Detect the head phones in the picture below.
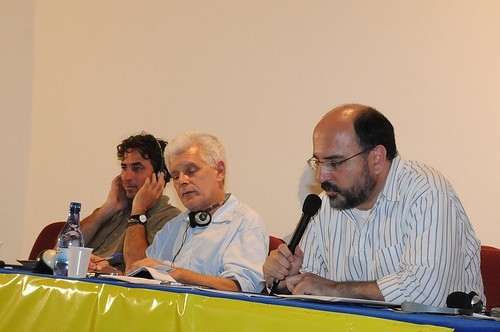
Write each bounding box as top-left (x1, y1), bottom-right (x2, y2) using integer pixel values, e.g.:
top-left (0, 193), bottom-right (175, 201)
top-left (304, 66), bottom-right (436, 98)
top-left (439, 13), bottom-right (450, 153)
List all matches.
top-left (186, 195), bottom-right (228, 228)
top-left (446, 291), bottom-right (484, 313)
top-left (156, 139), bottom-right (168, 180)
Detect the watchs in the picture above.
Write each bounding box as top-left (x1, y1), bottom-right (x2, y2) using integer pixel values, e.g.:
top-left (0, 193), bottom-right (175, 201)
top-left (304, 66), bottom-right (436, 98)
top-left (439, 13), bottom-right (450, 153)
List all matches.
top-left (129, 214), bottom-right (147, 223)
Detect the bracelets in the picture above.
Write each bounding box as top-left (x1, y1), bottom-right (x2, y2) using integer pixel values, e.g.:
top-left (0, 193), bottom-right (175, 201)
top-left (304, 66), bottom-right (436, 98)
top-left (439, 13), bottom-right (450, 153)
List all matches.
top-left (126, 222), bottom-right (146, 227)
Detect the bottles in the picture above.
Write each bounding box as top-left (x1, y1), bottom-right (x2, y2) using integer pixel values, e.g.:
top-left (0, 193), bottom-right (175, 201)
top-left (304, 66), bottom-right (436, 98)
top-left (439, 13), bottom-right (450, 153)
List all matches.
top-left (54, 202), bottom-right (86, 276)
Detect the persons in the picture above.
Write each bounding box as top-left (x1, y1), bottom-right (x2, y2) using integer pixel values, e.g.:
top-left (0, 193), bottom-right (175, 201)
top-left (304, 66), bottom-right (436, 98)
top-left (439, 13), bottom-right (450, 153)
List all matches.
top-left (53, 134), bottom-right (182, 272)
top-left (88, 131), bottom-right (270, 294)
top-left (262, 103), bottom-right (487, 310)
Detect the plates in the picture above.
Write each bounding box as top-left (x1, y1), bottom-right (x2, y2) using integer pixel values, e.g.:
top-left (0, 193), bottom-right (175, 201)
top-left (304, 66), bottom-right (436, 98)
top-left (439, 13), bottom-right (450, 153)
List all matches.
top-left (16, 260), bottom-right (36, 268)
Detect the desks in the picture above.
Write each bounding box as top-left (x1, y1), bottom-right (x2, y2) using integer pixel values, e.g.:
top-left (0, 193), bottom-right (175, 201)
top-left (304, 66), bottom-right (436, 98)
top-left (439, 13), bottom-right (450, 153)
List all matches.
top-left (0, 264), bottom-right (500, 332)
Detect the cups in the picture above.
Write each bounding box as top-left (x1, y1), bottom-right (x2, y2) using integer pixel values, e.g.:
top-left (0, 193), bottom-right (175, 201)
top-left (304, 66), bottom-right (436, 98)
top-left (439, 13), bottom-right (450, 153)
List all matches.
top-left (68, 246), bottom-right (94, 278)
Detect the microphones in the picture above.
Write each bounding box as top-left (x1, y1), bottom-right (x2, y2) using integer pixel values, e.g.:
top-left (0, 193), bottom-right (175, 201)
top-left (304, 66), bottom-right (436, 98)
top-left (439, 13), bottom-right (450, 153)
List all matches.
top-left (271, 194), bottom-right (322, 294)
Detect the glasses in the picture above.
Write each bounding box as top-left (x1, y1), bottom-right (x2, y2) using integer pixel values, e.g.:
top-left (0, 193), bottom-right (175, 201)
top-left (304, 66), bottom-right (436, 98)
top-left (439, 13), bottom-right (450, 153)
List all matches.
top-left (307, 146), bottom-right (376, 173)
